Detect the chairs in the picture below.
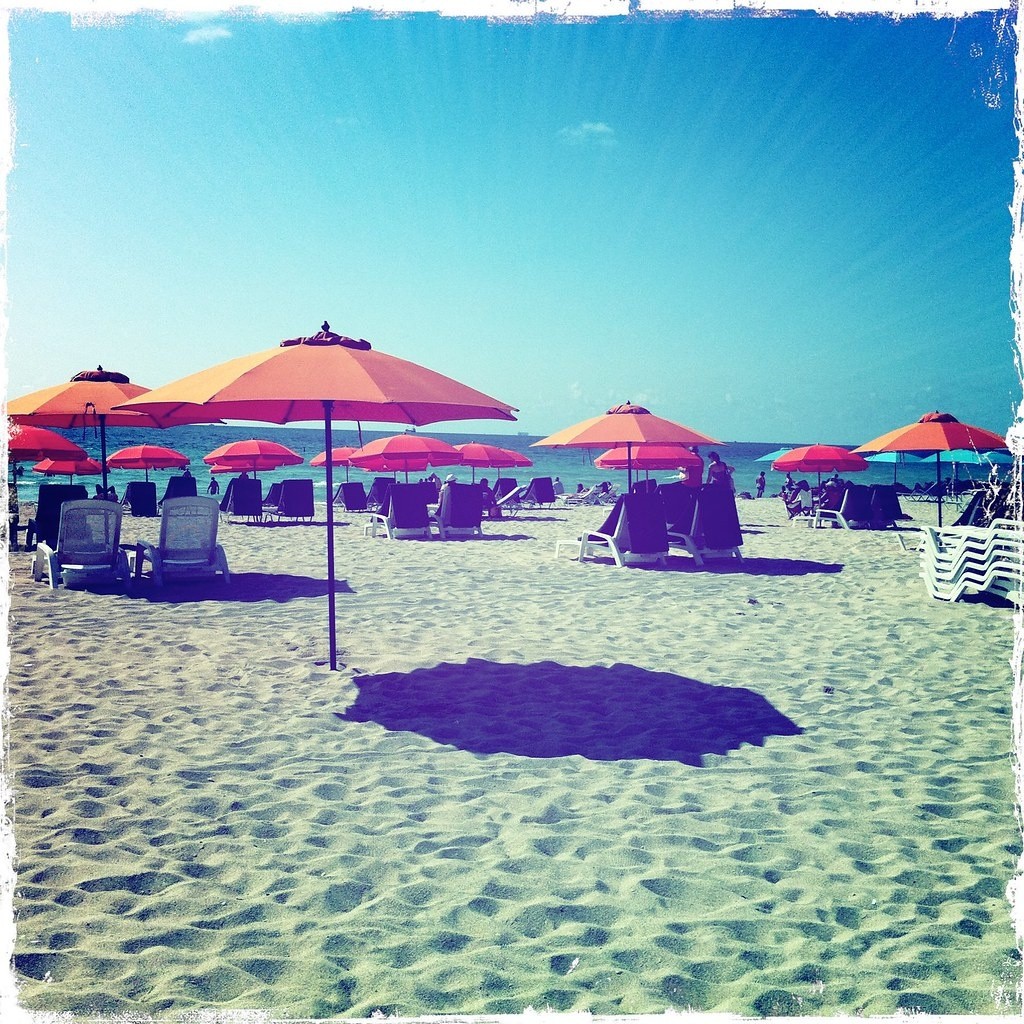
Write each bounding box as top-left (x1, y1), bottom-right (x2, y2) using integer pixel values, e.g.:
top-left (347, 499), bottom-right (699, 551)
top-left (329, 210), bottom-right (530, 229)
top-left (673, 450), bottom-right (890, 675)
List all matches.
top-left (261, 482), bottom-right (282, 507)
top-left (136, 496), bottom-right (231, 589)
top-left (792, 485), bottom-right (871, 528)
top-left (666, 483), bottom-right (745, 568)
top-left (364, 477), bottom-right (396, 512)
top-left (837, 485), bottom-right (897, 527)
top-left (427, 480), bottom-right (484, 540)
top-left (261, 478), bottom-right (315, 524)
top-left (895, 477), bottom-right (993, 512)
top-left (918, 518), bottom-right (1024, 607)
top-left (332, 482), bottom-right (369, 512)
top-left (482, 477), bottom-right (621, 519)
top-left (631, 479), bottom-right (657, 494)
top-left (34, 499), bottom-right (132, 592)
top-left (419, 482), bottom-right (438, 504)
top-left (119, 475), bottom-right (197, 517)
top-left (219, 478), bottom-right (262, 525)
top-left (25, 485), bottom-right (93, 552)
top-left (361, 482), bottom-right (439, 540)
top-left (555, 492), bottom-right (669, 569)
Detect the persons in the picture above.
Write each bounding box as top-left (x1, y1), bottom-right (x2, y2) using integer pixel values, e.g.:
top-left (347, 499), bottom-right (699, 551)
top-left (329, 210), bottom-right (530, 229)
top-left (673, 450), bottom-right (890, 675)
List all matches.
top-left (182, 467), bottom-right (191, 477)
top-left (756, 470), bottom-right (848, 528)
top-left (107, 486), bottom-right (118, 502)
top-left (682, 445), bottom-right (706, 488)
top-left (93, 484), bottom-right (105, 500)
top-left (239, 472), bottom-right (249, 479)
top-left (418, 472), bottom-right (612, 520)
top-left (705, 452), bottom-right (734, 484)
top-left (206, 477), bottom-right (220, 496)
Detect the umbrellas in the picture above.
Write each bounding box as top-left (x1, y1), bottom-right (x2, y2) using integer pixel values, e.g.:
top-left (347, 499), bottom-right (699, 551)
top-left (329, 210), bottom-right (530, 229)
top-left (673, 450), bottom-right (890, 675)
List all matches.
top-left (530, 402), bottom-right (729, 497)
top-left (772, 443), bottom-right (867, 510)
top-left (752, 446), bottom-right (798, 462)
top-left (8, 424), bottom-right (533, 496)
top-left (111, 319), bottom-right (519, 672)
top-left (594, 446), bottom-right (700, 495)
top-left (849, 411), bottom-right (1013, 527)
top-left (7, 363), bottom-right (226, 498)
